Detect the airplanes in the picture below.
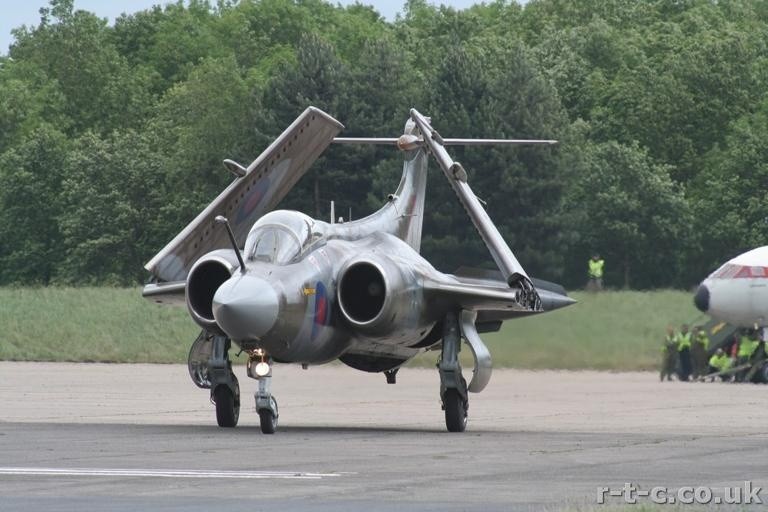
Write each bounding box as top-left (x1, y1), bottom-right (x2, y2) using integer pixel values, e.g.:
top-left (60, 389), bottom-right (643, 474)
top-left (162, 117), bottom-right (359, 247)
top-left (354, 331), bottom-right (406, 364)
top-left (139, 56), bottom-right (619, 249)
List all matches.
top-left (141, 105), bottom-right (580, 431)
top-left (690, 245), bottom-right (768, 327)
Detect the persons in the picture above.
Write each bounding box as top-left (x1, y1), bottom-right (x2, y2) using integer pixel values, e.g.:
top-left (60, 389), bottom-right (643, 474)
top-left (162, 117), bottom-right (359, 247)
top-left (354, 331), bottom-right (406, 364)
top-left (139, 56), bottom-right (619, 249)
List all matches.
top-left (587, 252), bottom-right (605, 290)
top-left (659, 323), bottom-right (767, 384)
